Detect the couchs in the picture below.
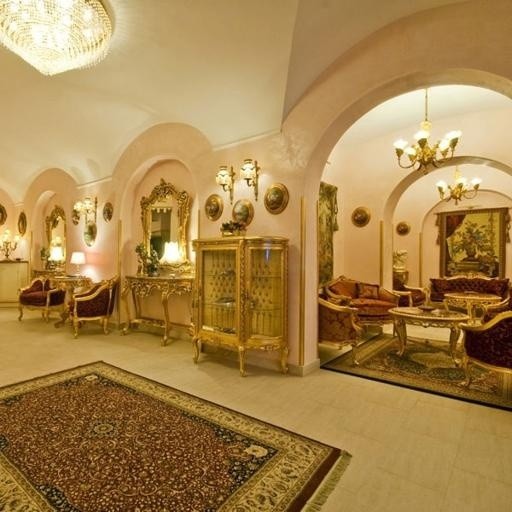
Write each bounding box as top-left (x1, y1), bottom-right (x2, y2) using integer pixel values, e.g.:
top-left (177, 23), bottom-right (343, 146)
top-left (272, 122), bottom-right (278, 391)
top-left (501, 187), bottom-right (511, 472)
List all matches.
top-left (17, 278), bottom-right (67, 324)
top-left (429, 275), bottom-right (510, 309)
top-left (318, 275), bottom-right (401, 367)
top-left (457, 311), bottom-right (512, 388)
top-left (393, 278), bottom-right (431, 307)
top-left (73, 274), bottom-right (119, 338)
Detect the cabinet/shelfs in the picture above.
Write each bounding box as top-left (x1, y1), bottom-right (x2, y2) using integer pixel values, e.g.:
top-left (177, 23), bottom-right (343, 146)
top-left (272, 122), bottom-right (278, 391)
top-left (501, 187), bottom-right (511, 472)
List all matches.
top-left (192, 236), bottom-right (289, 377)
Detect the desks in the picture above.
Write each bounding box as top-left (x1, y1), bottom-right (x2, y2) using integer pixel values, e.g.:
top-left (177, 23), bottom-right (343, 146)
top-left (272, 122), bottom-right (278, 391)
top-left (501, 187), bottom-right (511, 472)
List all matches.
top-left (121, 273), bottom-right (195, 347)
top-left (54, 276), bottom-right (92, 328)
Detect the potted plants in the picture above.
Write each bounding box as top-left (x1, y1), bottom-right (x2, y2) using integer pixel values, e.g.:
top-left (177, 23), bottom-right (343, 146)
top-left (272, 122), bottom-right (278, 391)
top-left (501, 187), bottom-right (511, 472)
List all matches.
top-left (135, 243), bottom-right (161, 276)
top-left (221, 219), bottom-right (247, 236)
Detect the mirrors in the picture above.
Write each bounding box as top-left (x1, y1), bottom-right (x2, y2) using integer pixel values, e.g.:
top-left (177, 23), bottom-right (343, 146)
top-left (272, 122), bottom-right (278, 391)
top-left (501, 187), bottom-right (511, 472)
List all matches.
top-left (140, 177), bottom-right (193, 274)
top-left (45, 204), bottom-right (67, 265)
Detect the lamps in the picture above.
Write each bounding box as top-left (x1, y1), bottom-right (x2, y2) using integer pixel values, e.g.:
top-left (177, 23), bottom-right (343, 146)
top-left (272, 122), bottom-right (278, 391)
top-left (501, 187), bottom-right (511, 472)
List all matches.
top-left (70, 251), bottom-right (86, 274)
top-left (392, 89), bottom-right (462, 175)
top-left (436, 165), bottom-right (482, 205)
top-left (74, 197), bottom-right (95, 224)
top-left (216, 165), bottom-right (236, 206)
top-left (0, 228), bottom-right (19, 260)
top-left (0, 0), bottom-right (113, 77)
top-left (240, 159), bottom-right (261, 202)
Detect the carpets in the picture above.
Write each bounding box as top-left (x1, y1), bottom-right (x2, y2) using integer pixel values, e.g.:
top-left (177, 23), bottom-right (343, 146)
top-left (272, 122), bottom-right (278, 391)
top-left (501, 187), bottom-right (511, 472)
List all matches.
top-left (320, 333), bottom-right (512, 412)
top-left (0, 360), bottom-right (353, 512)
